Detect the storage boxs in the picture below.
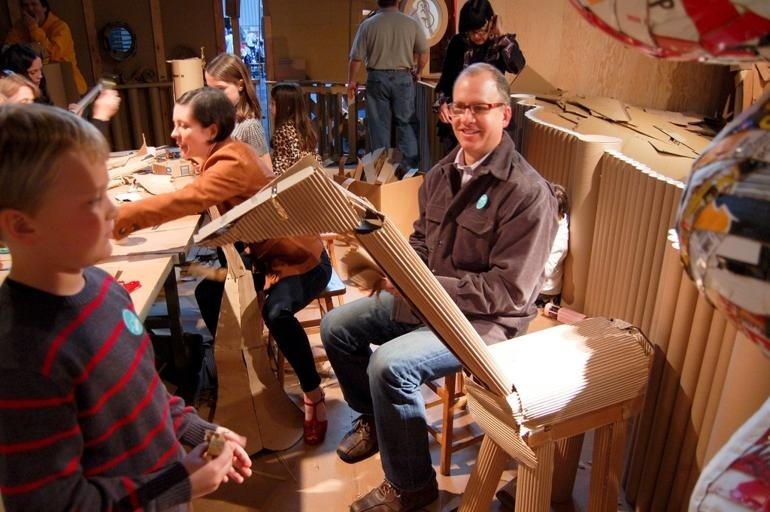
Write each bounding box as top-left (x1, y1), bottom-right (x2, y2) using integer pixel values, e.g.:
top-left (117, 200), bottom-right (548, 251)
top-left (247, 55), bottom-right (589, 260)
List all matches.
top-left (332, 165), bottom-right (423, 241)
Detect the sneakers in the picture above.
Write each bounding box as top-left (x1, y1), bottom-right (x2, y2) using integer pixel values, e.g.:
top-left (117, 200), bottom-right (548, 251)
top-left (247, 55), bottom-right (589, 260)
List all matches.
top-left (349, 475), bottom-right (439, 512)
top-left (337, 413), bottom-right (379, 464)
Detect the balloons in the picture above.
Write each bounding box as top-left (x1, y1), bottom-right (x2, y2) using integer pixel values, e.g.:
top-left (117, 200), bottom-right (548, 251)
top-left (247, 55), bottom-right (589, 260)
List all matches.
top-left (572, 1), bottom-right (770, 66)
top-left (687, 396), bottom-right (770, 512)
top-left (672, 83), bottom-right (770, 362)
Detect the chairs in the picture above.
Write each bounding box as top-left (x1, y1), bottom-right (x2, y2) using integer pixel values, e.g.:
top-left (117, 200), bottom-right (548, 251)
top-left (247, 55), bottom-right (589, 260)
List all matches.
top-left (209, 205), bottom-right (346, 392)
top-left (420, 181), bottom-right (569, 476)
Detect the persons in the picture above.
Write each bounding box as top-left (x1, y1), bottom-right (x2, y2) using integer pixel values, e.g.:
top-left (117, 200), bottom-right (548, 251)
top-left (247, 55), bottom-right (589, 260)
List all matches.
top-left (346, 0), bottom-right (428, 172)
top-left (0, 102), bottom-right (252, 510)
top-left (432, 0), bottom-right (525, 159)
top-left (319, 63), bottom-right (558, 512)
top-left (268, 83), bottom-right (318, 175)
top-left (205, 52), bottom-right (273, 174)
top-left (533, 183), bottom-right (569, 309)
top-left (0, 43), bottom-right (53, 105)
top-left (112, 86), bottom-right (331, 446)
top-left (0, 67), bottom-right (122, 146)
top-left (5, 0), bottom-right (88, 99)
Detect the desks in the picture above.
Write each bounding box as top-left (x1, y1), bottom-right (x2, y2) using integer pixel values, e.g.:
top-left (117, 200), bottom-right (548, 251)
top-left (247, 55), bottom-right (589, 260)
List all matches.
top-left (108, 150), bottom-right (206, 266)
top-left (0, 255), bottom-right (195, 406)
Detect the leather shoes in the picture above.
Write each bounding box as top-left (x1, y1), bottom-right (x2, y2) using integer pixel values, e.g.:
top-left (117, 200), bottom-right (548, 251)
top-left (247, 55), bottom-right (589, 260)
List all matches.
top-left (299, 386), bottom-right (328, 442)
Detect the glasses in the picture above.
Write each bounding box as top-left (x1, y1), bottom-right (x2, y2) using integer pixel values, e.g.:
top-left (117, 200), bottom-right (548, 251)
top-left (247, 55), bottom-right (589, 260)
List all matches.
top-left (447, 103), bottom-right (506, 120)
top-left (465, 21), bottom-right (490, 39)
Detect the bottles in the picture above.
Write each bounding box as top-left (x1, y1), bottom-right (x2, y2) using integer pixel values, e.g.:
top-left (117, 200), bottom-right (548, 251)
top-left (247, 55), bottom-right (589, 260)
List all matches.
top-left (543, 302), bottom-right (588, 324)
